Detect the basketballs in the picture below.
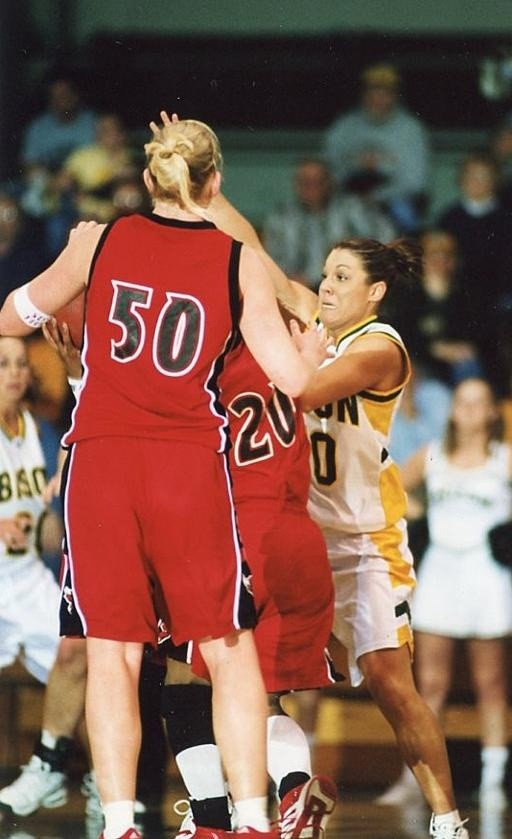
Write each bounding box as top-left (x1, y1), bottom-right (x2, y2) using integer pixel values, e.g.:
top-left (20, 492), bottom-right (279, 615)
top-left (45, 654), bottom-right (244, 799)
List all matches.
top-left (45, 287), bottom-right (87, 353)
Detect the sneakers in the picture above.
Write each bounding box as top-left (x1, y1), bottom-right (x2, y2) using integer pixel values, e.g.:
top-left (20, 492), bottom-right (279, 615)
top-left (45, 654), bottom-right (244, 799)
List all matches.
top-left (0, 755), bottom-right (70, 818)
top-left (429, 819), bottom-right (469, 839)
top-left (376, 784), bottom-right (423, 808)
top-left (173, 773), bottom-right (339, 839)
top-left (82, 771), bottom-right (148, 838)
top-left (479, 788), bottom-right (507, 812)
